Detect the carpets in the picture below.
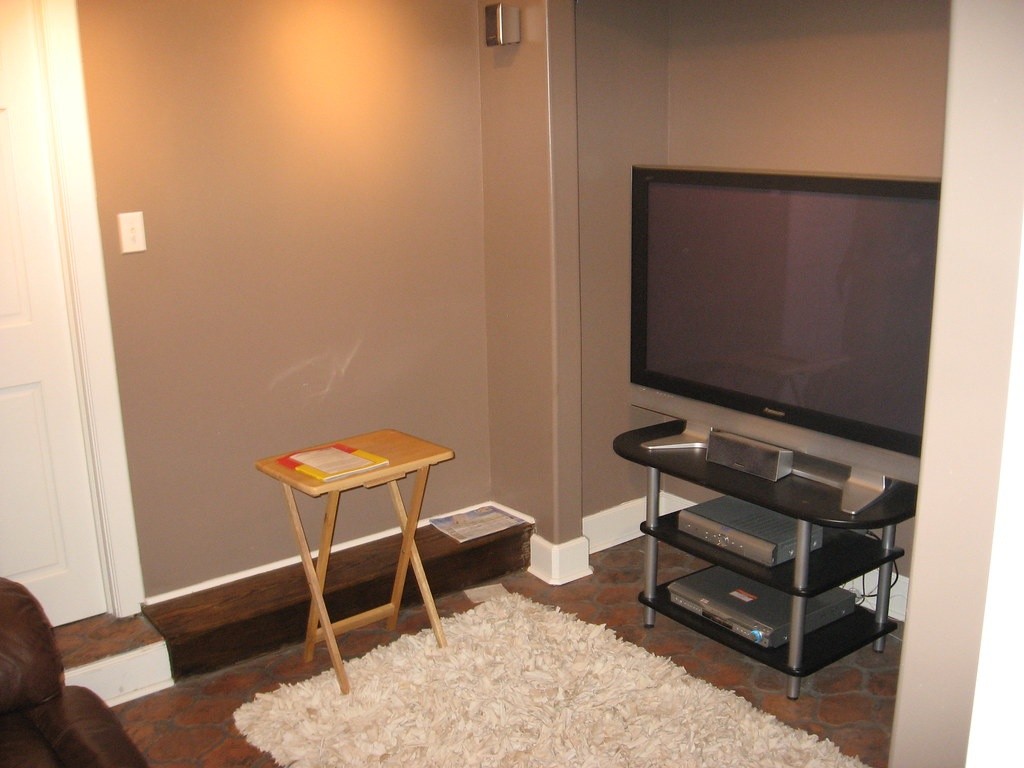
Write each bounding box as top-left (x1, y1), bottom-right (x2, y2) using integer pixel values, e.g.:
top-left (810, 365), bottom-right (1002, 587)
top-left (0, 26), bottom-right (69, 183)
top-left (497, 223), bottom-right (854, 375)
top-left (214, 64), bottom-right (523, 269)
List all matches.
top-left (232, 591), bottom-right (870, 768)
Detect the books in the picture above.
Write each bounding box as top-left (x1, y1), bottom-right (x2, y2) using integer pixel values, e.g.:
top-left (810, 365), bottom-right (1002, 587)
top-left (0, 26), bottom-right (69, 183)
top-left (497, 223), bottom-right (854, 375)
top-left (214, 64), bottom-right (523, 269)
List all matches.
top-left (277, 443), bottom-right (389, 484)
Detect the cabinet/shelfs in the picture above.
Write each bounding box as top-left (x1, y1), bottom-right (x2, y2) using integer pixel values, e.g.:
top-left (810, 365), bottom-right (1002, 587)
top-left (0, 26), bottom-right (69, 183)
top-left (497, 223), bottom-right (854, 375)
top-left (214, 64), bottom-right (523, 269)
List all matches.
top-left (613, 419), bottom-right (918, 702)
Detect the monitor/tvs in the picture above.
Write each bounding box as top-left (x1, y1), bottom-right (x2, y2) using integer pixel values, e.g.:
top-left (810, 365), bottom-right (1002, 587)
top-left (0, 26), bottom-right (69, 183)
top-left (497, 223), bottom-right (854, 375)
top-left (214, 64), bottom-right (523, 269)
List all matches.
top-left (629, 167), bottom-right (942, 515)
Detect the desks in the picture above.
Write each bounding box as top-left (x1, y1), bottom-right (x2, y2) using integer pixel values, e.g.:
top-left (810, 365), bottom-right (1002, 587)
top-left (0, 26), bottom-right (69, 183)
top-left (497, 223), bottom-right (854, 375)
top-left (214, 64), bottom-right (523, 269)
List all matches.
top-left (255, 428), bottom-right (455, 694)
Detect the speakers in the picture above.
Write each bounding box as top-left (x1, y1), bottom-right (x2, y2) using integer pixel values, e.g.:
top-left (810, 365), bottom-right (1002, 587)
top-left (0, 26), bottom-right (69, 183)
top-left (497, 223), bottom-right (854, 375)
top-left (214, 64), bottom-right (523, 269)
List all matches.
top-left (485, 3), bottom-right (520, 45)
top-left (705, 431), bottom-right (793, 482)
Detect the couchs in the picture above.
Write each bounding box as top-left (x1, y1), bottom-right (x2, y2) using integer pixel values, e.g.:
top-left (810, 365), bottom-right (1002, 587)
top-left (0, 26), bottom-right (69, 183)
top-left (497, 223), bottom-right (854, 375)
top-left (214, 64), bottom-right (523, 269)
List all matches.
top-left (0, 577), bottom-right (148, 768)
